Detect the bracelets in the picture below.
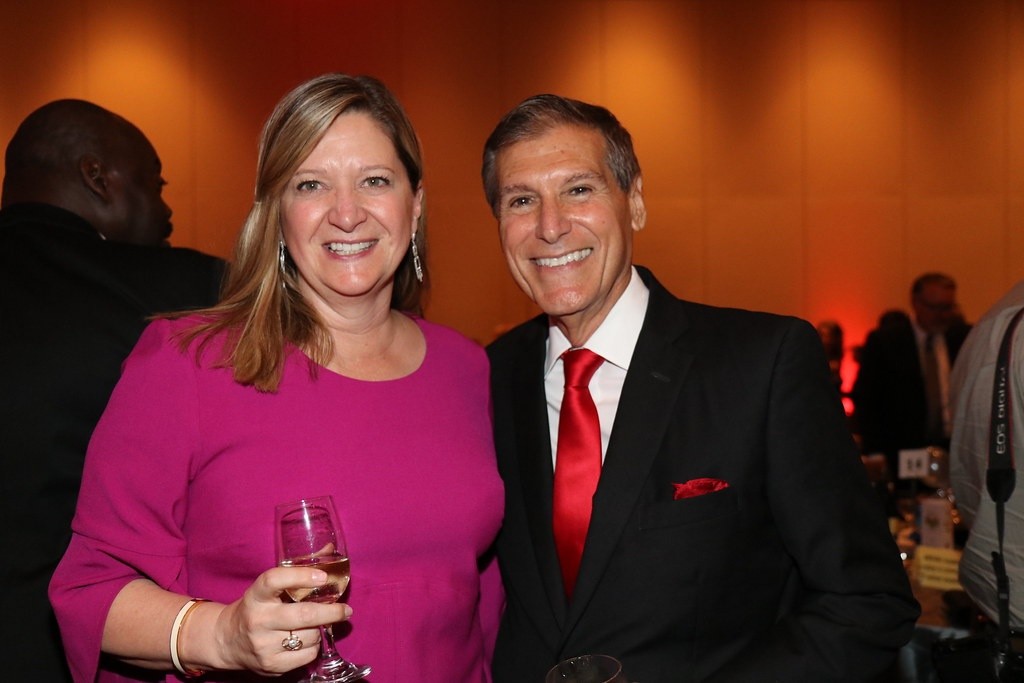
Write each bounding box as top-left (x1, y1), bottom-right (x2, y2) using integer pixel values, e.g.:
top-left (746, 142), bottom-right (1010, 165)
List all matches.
top-left (170, 598), bottom-right (213, 677)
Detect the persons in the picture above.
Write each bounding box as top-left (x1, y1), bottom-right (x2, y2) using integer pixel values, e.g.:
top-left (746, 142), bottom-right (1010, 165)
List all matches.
top-left (948, 281), bottom-right (1024, 683)
top-left (484, 93), bottom-right (921, 683)
top-left (0, 99), bottom-right (231, 683)
top-left (817, 271), bottom-right (973, 498)
top-left (48, 70), bottom-right (505, 683)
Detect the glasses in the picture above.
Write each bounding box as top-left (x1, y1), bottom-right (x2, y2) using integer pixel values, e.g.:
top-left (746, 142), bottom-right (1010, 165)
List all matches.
top-left (918, 298), bottom-right (957, 314)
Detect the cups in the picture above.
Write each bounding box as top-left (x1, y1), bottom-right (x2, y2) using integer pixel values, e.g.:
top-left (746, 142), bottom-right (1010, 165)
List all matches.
top-left (547, 654), bottom-right (622, 683)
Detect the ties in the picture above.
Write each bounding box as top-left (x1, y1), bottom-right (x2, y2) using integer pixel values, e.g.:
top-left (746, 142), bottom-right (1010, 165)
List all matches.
top-left (552, 348), bottom-right (606, 600)
top-left (925, 335), bottom-right (944, 424)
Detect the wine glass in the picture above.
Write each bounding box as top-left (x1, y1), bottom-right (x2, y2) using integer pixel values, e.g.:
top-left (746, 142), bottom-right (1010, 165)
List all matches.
top-left (275, 495), bottom-right (371, 682)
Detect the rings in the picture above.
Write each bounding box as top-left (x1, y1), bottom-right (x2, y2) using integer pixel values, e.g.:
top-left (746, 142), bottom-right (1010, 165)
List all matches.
top-left (282, 630), bottom-right (303, 651)
